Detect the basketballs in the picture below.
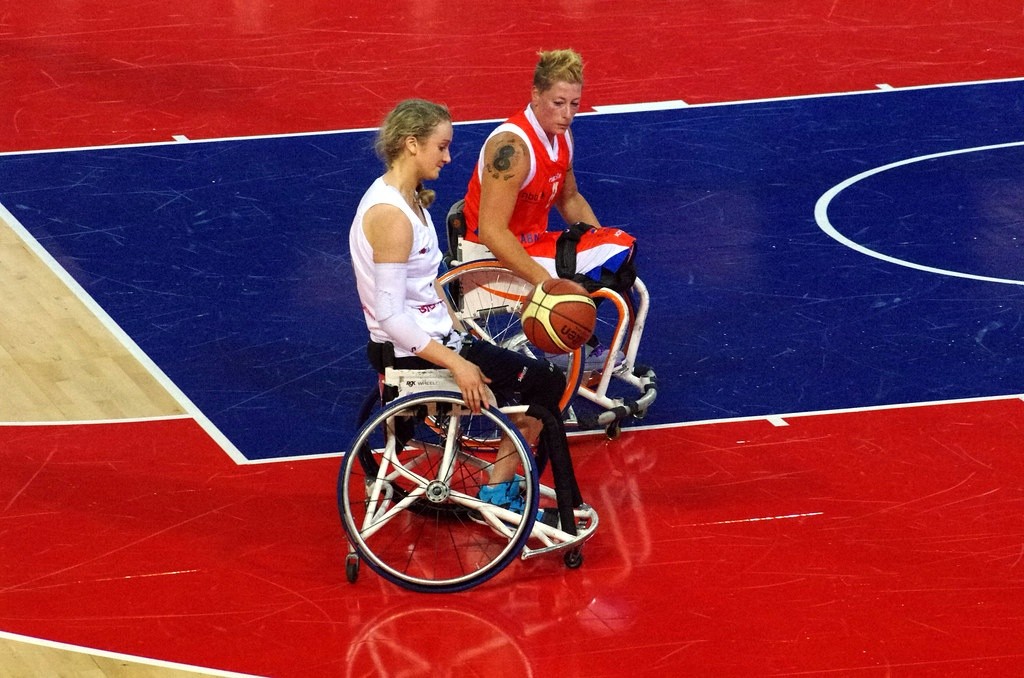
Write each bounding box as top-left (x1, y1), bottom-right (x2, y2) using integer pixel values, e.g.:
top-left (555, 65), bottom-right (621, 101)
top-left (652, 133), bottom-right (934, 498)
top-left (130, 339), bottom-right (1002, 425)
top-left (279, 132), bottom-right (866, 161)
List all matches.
top-left (520, 278), bottom-right (597, 354)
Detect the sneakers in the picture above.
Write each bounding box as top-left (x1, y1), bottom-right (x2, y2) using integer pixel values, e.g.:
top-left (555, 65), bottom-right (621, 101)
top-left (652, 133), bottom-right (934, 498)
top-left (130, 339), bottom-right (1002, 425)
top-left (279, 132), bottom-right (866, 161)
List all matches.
top-left (469, 475), bottom-right (549, 527)
top-left (544, 335), bottom-right (628, 372)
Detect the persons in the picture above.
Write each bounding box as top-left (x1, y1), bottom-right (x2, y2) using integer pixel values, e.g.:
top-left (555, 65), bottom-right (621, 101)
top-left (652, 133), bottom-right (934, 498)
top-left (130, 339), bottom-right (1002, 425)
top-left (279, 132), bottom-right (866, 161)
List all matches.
top-left (348, 100), bottom-right (566, 534)
top-left (461, 48), bottom-right (636, 371)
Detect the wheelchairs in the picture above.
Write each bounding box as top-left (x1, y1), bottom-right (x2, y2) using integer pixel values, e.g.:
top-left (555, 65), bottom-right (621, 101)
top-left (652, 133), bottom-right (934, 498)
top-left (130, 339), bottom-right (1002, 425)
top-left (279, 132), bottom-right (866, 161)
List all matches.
top-left (437, 200), bottom-right (660, 439)
top-left (336, 257), bottom-right (599, 594)
